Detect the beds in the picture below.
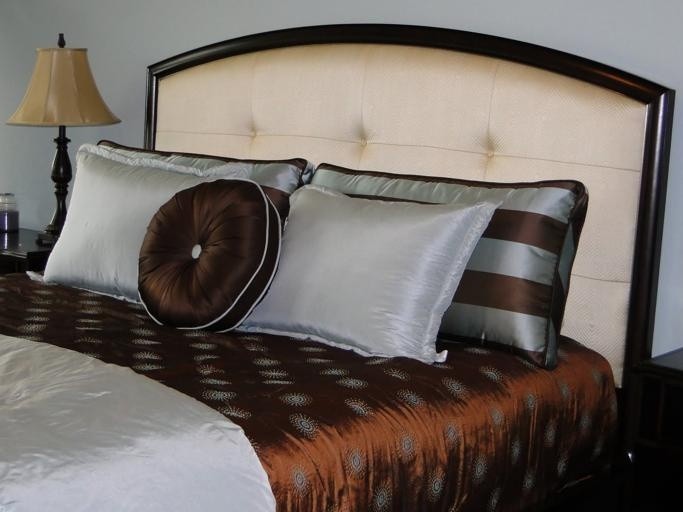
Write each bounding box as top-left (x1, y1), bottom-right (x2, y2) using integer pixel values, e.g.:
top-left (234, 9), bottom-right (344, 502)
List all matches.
top-left (0, 23), bottom-right (678, 511)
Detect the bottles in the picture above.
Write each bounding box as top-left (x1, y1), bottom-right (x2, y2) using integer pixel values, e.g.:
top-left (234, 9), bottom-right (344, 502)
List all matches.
top-left (0, 231), bottom-right (20, 253)
top-left (0, 192), bottom-right (19, 234)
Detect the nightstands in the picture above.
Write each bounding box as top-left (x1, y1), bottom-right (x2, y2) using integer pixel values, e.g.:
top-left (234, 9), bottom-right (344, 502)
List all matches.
top-left (0, 228), bottom-right (56, 273)
top-left (610, 348), bottom-right (682, 512)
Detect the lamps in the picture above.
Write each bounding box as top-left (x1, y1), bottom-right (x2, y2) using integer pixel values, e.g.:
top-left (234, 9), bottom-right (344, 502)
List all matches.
top-left (6, 30), bottom-right (120, 247)
